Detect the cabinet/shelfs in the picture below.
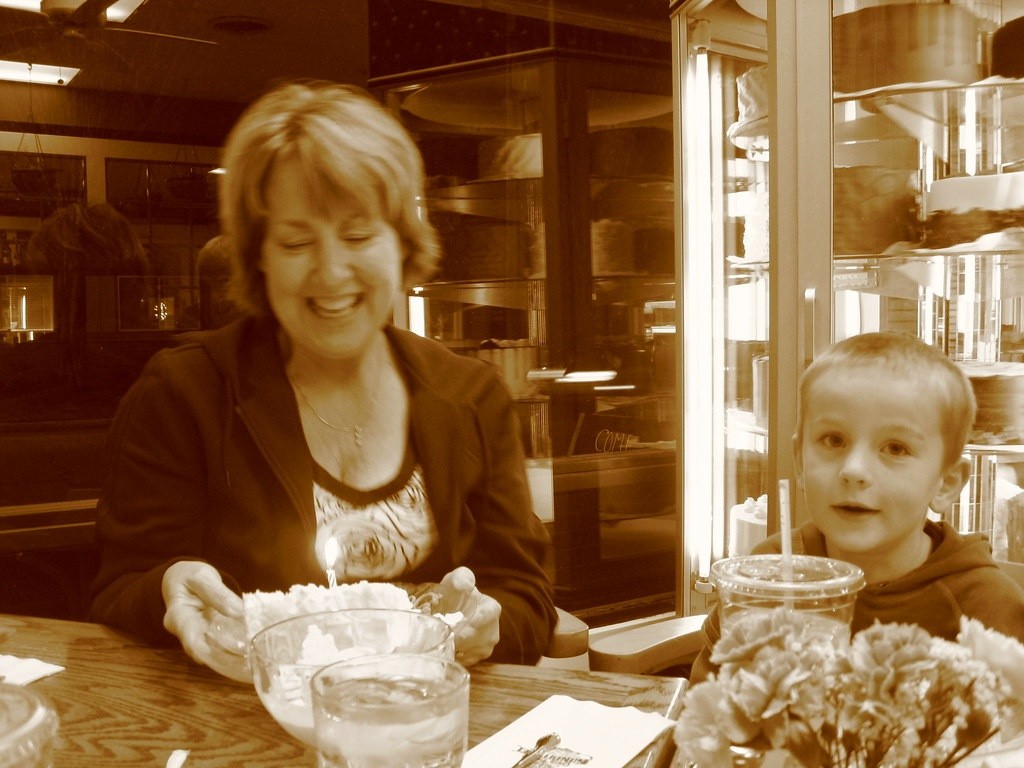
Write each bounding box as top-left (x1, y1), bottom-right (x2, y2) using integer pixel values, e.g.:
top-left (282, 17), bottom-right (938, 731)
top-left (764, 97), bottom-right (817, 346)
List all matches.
top-left (668, 2), bottom-right (1024, 608)
top-left (367, 45), bottom-right (670, 614)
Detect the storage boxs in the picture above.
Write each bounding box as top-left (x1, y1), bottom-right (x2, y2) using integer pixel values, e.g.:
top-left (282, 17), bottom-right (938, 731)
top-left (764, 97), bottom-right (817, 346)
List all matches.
top-left (835, 3), bottom-right (981, 93)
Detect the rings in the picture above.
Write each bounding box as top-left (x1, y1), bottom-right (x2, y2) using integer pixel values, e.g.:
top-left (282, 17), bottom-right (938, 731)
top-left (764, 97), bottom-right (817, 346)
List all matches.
top-left (456, 650), bottom-right (465, 658)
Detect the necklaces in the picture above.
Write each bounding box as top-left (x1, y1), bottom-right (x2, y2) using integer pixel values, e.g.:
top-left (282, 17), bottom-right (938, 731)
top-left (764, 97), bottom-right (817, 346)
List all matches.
top-left (289, 335), bottom-right (382, 447)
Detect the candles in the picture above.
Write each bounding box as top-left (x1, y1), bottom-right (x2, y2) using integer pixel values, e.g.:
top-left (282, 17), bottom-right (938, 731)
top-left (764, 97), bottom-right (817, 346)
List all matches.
top-left (325, 570), bottom-right (342, 610)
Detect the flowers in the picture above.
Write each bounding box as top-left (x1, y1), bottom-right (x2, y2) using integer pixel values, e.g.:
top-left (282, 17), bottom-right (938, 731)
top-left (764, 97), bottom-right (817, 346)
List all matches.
top-left (670, 609), bottom-right (1024, 768)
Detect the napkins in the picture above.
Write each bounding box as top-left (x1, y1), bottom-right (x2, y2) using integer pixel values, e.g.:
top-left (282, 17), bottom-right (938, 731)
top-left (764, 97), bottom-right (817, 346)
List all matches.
top-left (461, 695), bottom-right (677, 768)
top-left (0, 654), bottom-right (66, 686)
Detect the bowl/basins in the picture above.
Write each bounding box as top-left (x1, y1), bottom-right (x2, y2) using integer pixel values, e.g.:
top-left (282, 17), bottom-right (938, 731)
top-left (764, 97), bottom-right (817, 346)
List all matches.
top-left (247, 607), bottom-right (457, 748)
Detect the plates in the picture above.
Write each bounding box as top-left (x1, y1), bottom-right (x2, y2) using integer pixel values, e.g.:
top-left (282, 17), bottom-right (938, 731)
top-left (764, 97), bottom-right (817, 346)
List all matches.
top-left (201, 580), bottom-right (483, 686)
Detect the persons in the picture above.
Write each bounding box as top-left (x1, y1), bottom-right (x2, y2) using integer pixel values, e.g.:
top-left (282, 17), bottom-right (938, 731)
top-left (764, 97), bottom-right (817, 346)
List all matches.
top-left (689, 331), bottom-right (1024, 693)
top-left (171, 236), bottom-right (249, 345)
top-left (85, 85), bottom-right (556, 683)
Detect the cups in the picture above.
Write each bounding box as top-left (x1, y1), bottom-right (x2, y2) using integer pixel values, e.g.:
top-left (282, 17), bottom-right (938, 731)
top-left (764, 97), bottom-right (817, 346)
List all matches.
top-left (0, 683), bottom-right (64, 768)
top-left (309, 655), bottom-right (471, 768)
top-left (708, 555), bottom-right (867, 649)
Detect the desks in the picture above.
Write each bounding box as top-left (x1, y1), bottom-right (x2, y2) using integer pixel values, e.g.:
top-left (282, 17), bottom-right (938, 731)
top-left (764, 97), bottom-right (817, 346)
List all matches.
top-left (0, 615), bottom-right (693, 768)
